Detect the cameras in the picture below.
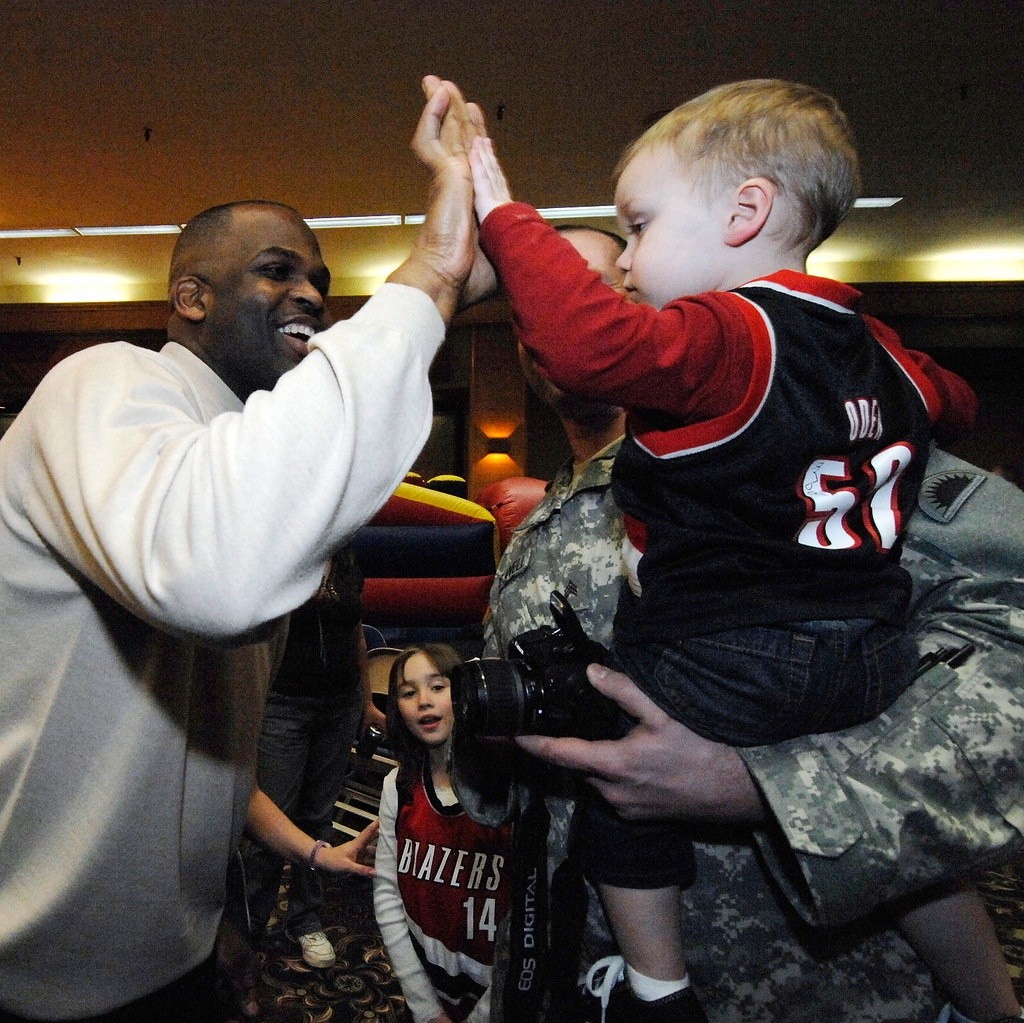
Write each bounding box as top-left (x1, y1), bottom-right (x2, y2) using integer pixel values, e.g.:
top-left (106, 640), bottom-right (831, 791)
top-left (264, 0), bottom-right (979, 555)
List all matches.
top-left (451, 590), bottom-right (629, 744)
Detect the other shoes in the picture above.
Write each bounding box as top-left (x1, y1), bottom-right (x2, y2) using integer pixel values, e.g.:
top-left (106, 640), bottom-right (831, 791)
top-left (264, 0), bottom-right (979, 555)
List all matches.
top-left (547, 952), bottom-right (709, 1023)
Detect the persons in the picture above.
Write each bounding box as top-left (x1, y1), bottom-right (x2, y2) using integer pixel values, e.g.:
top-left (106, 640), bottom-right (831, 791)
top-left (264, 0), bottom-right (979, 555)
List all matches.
top-left (468, 78), bottom-right (1024, 1023)
top-left (372, 642), bottom-right (515, 1023)
top-left (1, 75), bottom-right (498, 1023)
top-left (485, 223), bottom-right (1023, 1023)
top-left (244, 787), bottom-right (379, 879)
top-left (244, 548), bottom-right (385, 968)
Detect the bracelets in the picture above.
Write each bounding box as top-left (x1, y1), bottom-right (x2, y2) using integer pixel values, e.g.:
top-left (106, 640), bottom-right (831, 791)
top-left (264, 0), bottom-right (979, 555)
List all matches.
top-left (310, 840), bottom-right (331, 870)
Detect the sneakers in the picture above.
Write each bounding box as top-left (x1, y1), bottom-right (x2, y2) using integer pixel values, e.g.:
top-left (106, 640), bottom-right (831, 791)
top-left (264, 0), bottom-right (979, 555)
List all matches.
top-left (286, 924), bottom-right (336, 968)
